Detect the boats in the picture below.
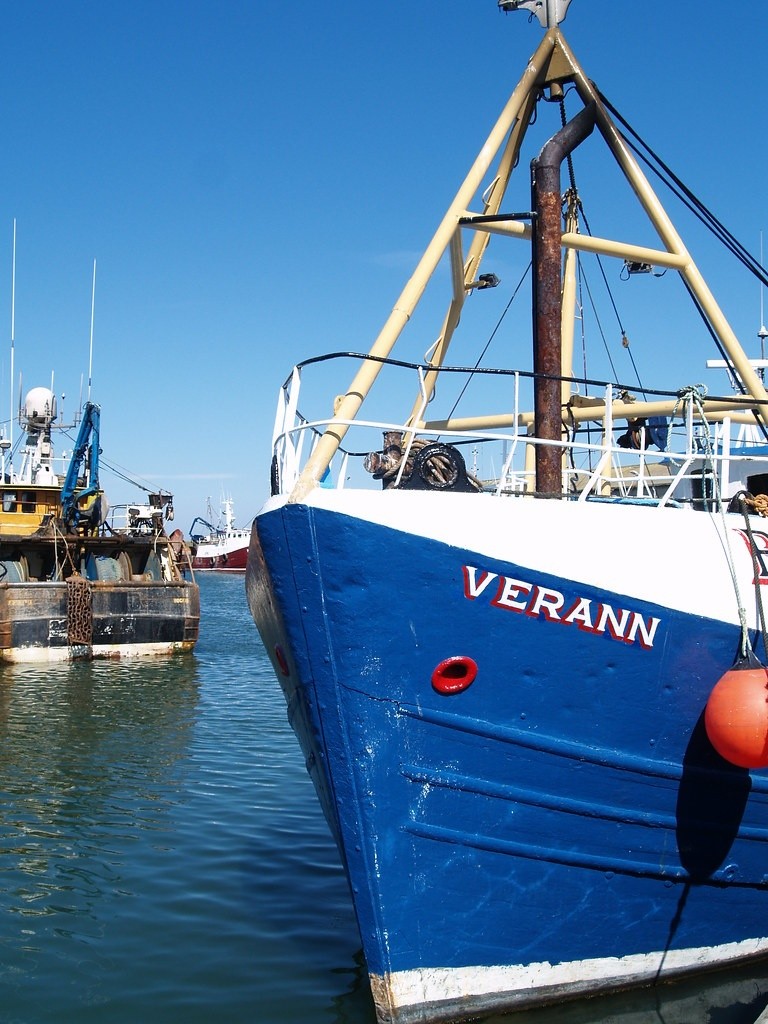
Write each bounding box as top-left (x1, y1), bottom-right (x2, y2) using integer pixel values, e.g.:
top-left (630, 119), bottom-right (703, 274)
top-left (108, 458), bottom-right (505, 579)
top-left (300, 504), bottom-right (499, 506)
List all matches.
top-left (245, 0), bottom-right (768, 1024)
top-left (0, 219), bottom-right (201, 663)
top-left (182, 494), bottom-right (251, 570)
